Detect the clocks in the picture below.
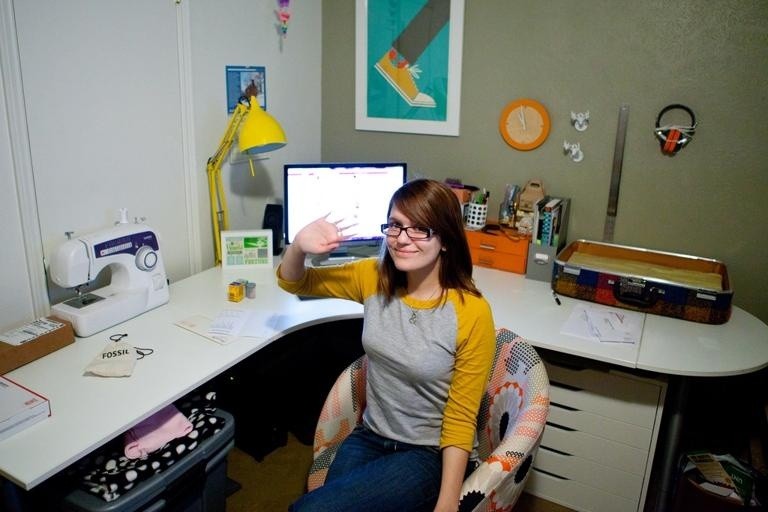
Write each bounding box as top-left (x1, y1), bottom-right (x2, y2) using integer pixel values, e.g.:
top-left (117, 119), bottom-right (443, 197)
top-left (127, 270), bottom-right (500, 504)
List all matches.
top-left (500, 99), bottom-right (550, 150)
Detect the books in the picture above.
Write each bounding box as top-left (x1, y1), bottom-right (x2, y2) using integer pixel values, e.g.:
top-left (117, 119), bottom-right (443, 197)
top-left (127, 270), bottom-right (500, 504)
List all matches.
top-left (533, 195), bottom-right (566, 246)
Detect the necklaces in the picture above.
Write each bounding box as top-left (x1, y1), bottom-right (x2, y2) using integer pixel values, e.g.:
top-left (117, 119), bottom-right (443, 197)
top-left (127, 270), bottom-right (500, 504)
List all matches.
top-left (397, 282), bottom-right (440, 323)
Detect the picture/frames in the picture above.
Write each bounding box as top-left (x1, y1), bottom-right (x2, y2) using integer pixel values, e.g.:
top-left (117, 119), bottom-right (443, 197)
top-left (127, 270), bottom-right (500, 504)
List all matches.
top-left (355, 0), bottom-right (464, 136)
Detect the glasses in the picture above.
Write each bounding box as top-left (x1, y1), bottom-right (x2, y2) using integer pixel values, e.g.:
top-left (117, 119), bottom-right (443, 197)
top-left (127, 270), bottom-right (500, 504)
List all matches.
top-left (378, 221), bottom-right (440, 241)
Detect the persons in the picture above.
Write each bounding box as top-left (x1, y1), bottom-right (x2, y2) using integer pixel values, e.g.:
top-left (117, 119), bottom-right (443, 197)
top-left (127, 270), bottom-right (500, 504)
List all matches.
top-left (274, 178), bottom-right (497, 512)
top-left (374, 0), bottom-right (450, 109)
top-left (244, 77), bottom-right (259, 98)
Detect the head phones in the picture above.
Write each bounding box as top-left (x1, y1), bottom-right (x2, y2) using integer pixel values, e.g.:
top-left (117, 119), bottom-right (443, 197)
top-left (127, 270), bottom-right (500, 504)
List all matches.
top-left (656, 104), bottom-right (696, 156)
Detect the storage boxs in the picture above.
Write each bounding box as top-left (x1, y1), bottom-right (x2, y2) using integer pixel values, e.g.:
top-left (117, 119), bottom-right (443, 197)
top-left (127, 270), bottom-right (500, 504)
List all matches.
top-left (50, 393), bottom-right (235, 512)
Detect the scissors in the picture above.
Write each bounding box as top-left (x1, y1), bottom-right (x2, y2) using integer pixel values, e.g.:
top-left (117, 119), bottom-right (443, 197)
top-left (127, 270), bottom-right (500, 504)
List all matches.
top-left (498, 222), bottom-right (527, 242)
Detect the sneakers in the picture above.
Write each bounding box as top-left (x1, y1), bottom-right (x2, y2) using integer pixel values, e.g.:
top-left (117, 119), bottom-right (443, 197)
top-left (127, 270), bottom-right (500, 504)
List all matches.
top-left (371, 43), bottom-right (441, 112)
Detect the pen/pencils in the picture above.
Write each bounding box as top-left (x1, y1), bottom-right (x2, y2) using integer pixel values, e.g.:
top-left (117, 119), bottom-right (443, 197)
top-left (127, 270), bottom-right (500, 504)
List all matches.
top-left (451, 185), bottom-right (480, 190)
top-left (552, 290), bottom-right (560, 305)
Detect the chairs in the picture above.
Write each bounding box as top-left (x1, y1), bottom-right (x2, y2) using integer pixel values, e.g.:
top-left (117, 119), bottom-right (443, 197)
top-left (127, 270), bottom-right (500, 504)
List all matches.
top-left (307, 328), bottom-right (550, 512)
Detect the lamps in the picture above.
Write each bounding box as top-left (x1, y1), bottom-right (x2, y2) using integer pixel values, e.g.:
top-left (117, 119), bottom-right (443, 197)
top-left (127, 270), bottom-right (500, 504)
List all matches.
top-left (206, 80), bottom-right (287, 268)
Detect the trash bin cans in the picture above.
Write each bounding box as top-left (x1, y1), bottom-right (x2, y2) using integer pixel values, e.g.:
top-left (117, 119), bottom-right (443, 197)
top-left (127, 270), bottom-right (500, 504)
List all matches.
top-left (668, 450), bottom-right (768, 511)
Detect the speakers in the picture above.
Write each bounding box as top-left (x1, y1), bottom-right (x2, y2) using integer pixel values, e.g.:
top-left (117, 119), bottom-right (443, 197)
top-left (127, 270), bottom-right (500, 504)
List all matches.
top-left (262, 204), bottom-right (283, 255)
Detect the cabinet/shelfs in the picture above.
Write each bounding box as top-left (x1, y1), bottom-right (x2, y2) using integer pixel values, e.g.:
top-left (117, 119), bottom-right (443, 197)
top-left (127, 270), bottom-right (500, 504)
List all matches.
top-left (522, 358), bottom-right (667, 511)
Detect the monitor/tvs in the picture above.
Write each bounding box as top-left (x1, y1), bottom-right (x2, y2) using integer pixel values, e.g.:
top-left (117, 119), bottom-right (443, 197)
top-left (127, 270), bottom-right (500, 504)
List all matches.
top-left (284, 162), bottom-right (407, 266)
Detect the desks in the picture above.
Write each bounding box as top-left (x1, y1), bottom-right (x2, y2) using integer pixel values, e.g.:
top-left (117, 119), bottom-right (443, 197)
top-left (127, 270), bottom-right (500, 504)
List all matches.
top-left (0, 258), bottom-right (767, 492)
